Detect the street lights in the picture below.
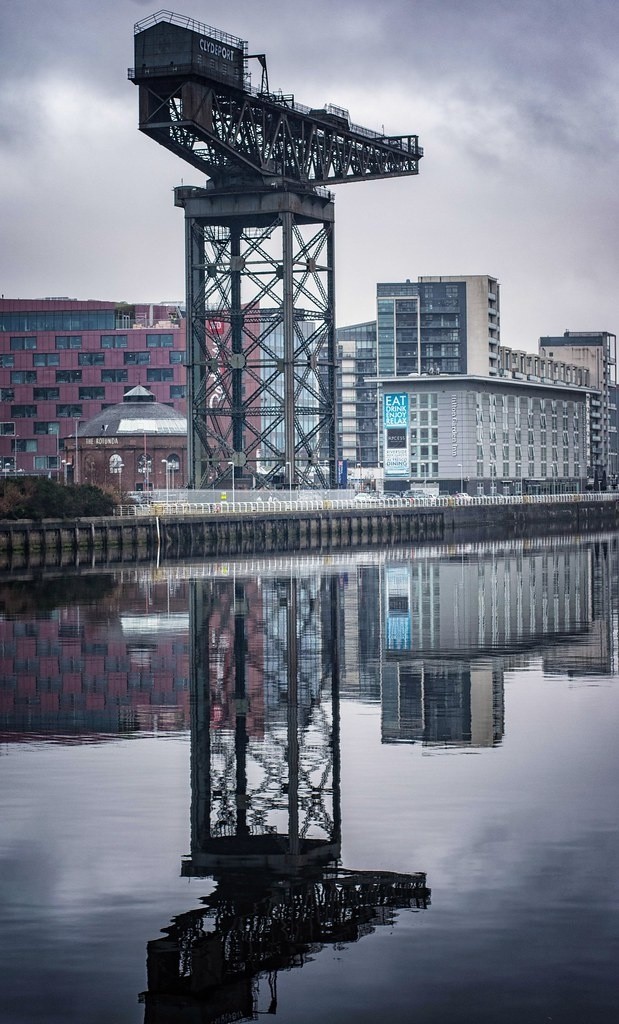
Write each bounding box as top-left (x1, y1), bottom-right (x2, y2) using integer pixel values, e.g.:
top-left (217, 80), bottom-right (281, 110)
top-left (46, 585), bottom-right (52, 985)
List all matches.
top-left (488, 464), bottom-right (494, 496)
top-left (61, 459), bottom-right (72, 487)
top-left (420, 462), bottom-right (428, 498)
top-left (517, 464), bottom-right (523, 496)
top-left (161, 458), bottom-right (170, 504)
top-left (118, 463), bottom-right (124, 491)
top-left (457, 464), bottom-right (464, 492)
top-left (286, 461), bottom-right (291, 501)
top-left (4, 463), bottom-right (10, 479)
top-left (578, 465), bottom-right (582, 494)
top-left (550, 465), bottom-right (554, 494)
top-left (357, 463), bottom-right (363, 493)
top-left (228, 461), bottom-right (235, 502)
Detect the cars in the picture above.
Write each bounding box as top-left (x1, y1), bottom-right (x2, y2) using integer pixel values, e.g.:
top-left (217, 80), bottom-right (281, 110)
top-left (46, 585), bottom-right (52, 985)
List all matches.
top-left (350, 487), bottom-right (505, 502)
